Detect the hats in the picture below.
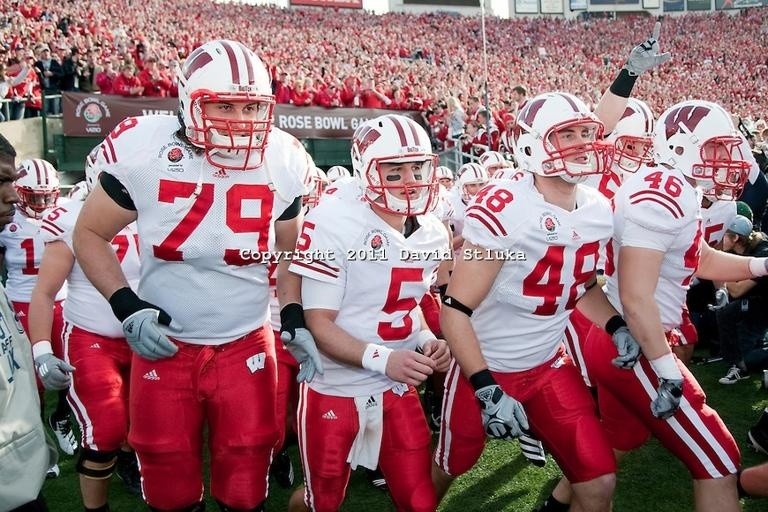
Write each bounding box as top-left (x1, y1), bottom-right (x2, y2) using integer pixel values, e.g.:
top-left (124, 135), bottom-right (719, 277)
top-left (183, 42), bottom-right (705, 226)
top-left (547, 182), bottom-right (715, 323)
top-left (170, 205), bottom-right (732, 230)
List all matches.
top-left (727, 214), bottom-right (753, 236)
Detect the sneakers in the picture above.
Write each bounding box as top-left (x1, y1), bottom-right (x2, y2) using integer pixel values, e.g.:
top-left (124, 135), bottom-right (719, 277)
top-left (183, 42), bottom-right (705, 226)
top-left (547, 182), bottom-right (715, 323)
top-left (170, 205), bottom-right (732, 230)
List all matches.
top-left (46, 464), bottom-right (59, 479)
top-left (275, 449), bottom-right (294, 488)
top-left (719, 364), bottom-right (752, 383)
top-left (50, 409), bottom-right (78, 456)
top-left (520, 429), bottom-right (548, 467)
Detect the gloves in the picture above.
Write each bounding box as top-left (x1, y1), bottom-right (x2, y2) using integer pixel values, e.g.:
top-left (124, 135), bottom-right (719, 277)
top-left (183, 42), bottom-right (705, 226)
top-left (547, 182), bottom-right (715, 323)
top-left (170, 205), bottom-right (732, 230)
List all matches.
top-left (278, 302), bottom-right (325, 383)
top-left (707, 287), bottom-right (729, 310)
top-left (606, 313), bottom-right (642, 371)
top-left (608, 21), bottom-right (670, 99)
top-left (651, 354), bottom-right (685, 419)
top-left (108, 287), bottom-right (183, 362)
top-left (751, 256), bottom-right (768, 276)
top-left (469, 368), bottom-right (530, 439)
top-left (31, 340), bottom-right (77, 395)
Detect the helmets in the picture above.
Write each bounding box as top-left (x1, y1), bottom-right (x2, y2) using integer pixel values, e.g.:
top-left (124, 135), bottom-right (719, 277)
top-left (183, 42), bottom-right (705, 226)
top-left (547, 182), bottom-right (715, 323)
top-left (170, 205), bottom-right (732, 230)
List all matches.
top-left (179, 40), bottom-right (273, 159)
top-left (649, 100), bottom-right (735, 182)
top-left (67, 182), bottom-right (87, 200)
top-left (351, 114), bottom-right (433, 215)
top-left (512, 92), bottom-right (602, 183)
top-left (456, 163), bottom-right (488, 185)
top-left (13, 159), bottom-right (60, 220)
top-left (434, 167), bottom-right (449, 180)
top-left (436, 166), bottom-right (453, 181)
top-left (608, 98), bottom-right (654, 168)
top-left (86, 141), bottom-right (106, 192)
top-left (328, 167), bottom-right (350, 183)
top-left (726, 131), bottom-right (760, 185)
top-left (478, 152), bottom-right (509, 168)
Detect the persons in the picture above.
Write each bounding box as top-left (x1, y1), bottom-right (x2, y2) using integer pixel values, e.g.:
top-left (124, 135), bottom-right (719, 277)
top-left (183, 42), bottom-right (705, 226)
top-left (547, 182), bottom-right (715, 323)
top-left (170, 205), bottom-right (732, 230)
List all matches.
top-left (0, 0), bottom-right (768, 147)
top-left (536, 99), bottom-right (768, 512)
top-left (268, 151), bottom-right (321, 490)
top-left (672, 128), bottom-right (760, 365)
top-left (432, 92), bottom-right (617, 512)
top-left (72, 39), bottom-right (323, 511)
top-left (28, 141), bottom-right (139, 511)
top-left (429, 182), bottom-right (453, 299)
top-left (1, 159), bottom-right (80, 457)
top-left (479, 150), bottom-right (506, 179)
top-left (435, 166), bottom-right (454, 189)
top-left (0, 132), bottom-right (58, 511)
top-left (593, 22), bottom-right (671, 210)
top-left (715, 214), bottom-right (768, 384)
top-left (448, 163), bottom-right (487, 277)
top-left (289, 115), bottom-right (452, 512)
